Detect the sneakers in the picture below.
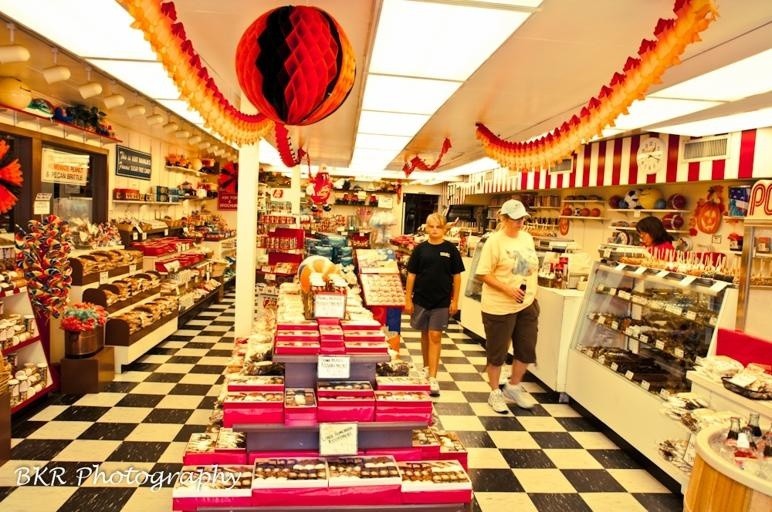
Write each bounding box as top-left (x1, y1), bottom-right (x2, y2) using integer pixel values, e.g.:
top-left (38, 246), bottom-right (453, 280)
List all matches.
top-left (488, 389), bottom-right (509, 414)
top-left (503, 380), bottom-right (534, 409)
top-left (421, 366), bottom-right (439, 394)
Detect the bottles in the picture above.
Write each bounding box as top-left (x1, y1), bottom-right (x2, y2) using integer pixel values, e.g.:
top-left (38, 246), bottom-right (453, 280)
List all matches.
top-left (727, 412), bottom-right (772, 469)
top-left (516, 278), bottom-right (526, 304)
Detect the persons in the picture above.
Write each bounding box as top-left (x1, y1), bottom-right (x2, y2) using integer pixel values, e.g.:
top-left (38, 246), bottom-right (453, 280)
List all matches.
top-left (404, 213), bottom-right (466, 392)
top-left (635, 216), bottom-right (678, 263)
top-left (474, 199), bottom-right (541, 414)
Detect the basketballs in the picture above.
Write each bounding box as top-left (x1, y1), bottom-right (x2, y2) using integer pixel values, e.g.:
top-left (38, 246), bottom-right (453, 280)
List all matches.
top-left (298, 255), bottom-right (339, 294)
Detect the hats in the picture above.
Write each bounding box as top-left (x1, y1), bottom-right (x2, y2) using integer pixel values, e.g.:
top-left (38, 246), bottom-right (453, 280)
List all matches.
top-left (499, 200), bottom-right (531, 220)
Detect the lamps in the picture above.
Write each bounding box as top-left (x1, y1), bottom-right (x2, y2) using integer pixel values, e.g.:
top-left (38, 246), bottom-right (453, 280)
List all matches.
top-left (348, 0), bottom-right (549, 172)
top-left (0, 0), bottom-right (239, 163)
